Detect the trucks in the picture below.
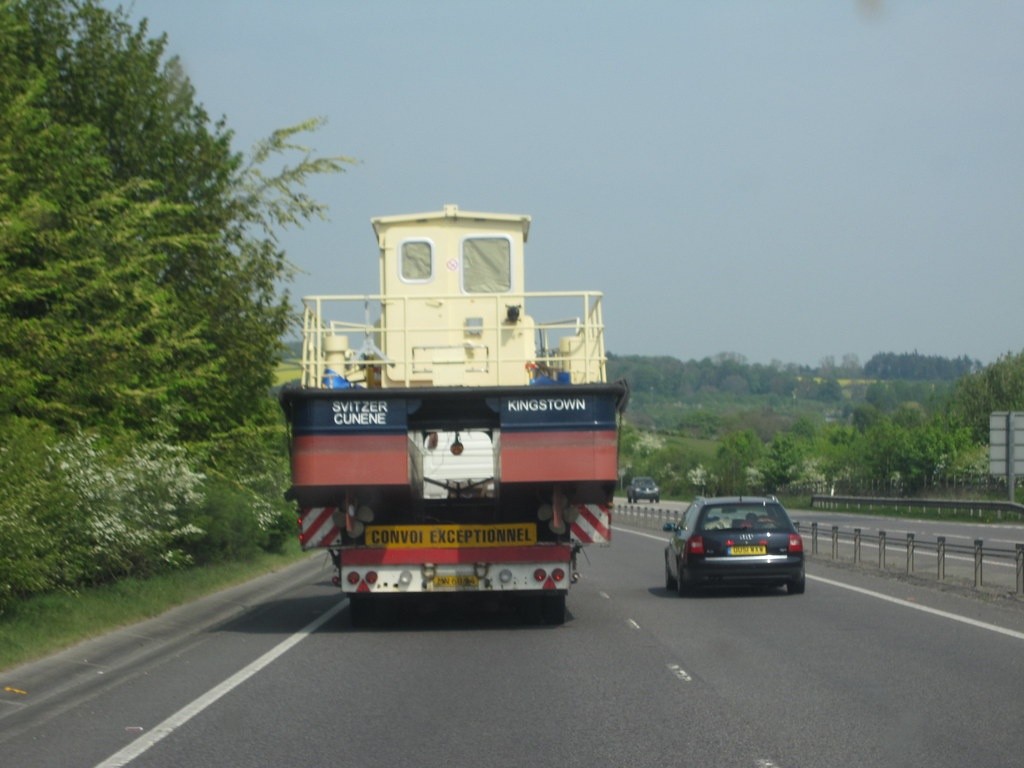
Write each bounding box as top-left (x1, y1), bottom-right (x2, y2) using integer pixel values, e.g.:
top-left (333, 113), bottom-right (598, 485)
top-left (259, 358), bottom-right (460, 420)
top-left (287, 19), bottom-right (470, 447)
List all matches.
top-left (280, 204), bottom-right (630, 624)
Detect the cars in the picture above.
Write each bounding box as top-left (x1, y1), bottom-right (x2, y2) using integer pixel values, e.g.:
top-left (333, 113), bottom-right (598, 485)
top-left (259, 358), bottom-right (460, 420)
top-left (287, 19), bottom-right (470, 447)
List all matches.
top-left (628, 476), bottom-right (661, 504)
top-left (661, 495), bottom-right (806, 596)
